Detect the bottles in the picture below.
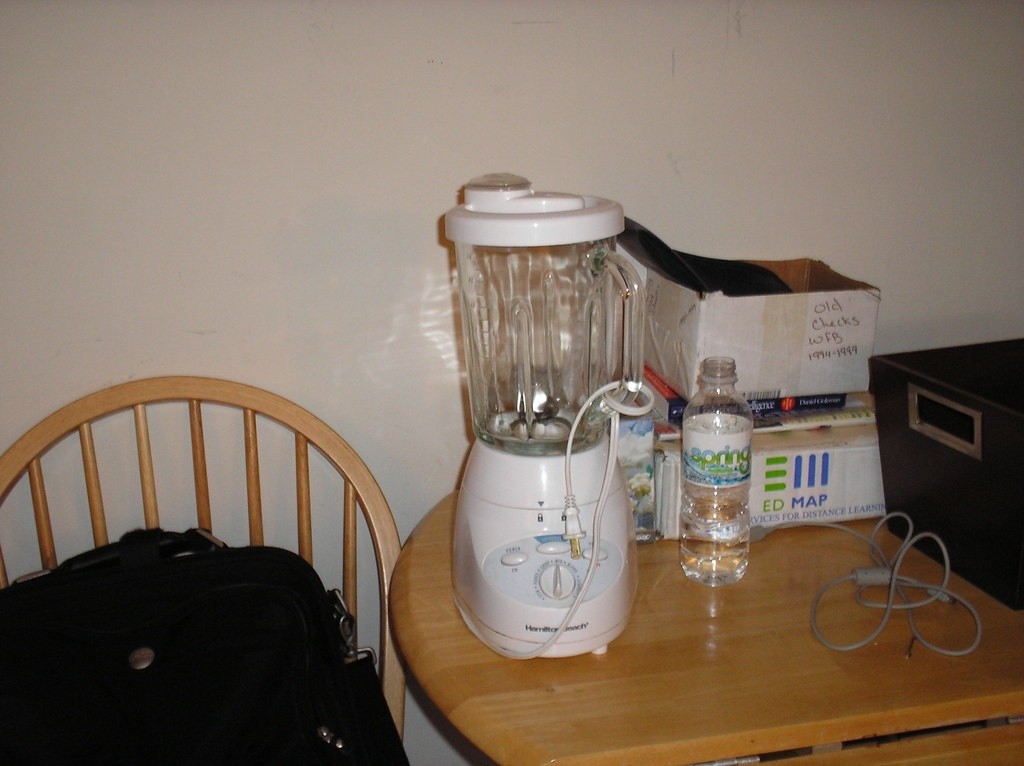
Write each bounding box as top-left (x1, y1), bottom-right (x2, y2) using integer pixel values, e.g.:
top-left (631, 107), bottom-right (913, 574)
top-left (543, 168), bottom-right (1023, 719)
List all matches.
top-left (681, 356), bottom-right (751, 588)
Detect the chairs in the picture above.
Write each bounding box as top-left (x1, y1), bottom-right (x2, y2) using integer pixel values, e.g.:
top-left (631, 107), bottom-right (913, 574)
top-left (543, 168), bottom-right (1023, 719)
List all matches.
top-left (0, 373), bottom-right (405, 750)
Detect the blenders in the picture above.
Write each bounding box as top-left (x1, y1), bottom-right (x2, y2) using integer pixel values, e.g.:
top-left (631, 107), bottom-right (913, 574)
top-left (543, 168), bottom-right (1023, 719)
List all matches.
top-left (444, 173), bottom-right (654, 659)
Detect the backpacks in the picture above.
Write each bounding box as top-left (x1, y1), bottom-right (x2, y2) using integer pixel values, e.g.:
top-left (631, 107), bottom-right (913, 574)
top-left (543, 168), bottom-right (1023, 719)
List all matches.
top-left (0, 528), bottom-right (410, 766)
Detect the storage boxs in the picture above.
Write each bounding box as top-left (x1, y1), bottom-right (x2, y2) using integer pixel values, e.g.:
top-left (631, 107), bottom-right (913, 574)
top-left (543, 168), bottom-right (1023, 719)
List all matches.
top-left (652, 424), bottom-right (887, 537)
top-left (634, 255), bottom-right (880, 402)
top-left (870, 337), bottom-right (1024, 613)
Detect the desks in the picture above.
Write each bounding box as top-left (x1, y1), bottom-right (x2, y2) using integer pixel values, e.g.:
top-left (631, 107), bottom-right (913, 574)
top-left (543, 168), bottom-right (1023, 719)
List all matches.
top-left (386, 483), bottom-right (1023, 765)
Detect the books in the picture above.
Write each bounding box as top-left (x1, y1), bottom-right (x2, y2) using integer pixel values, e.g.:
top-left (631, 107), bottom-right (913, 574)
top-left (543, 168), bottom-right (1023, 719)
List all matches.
top-left (643, 364), bottom-right (877, 441)
